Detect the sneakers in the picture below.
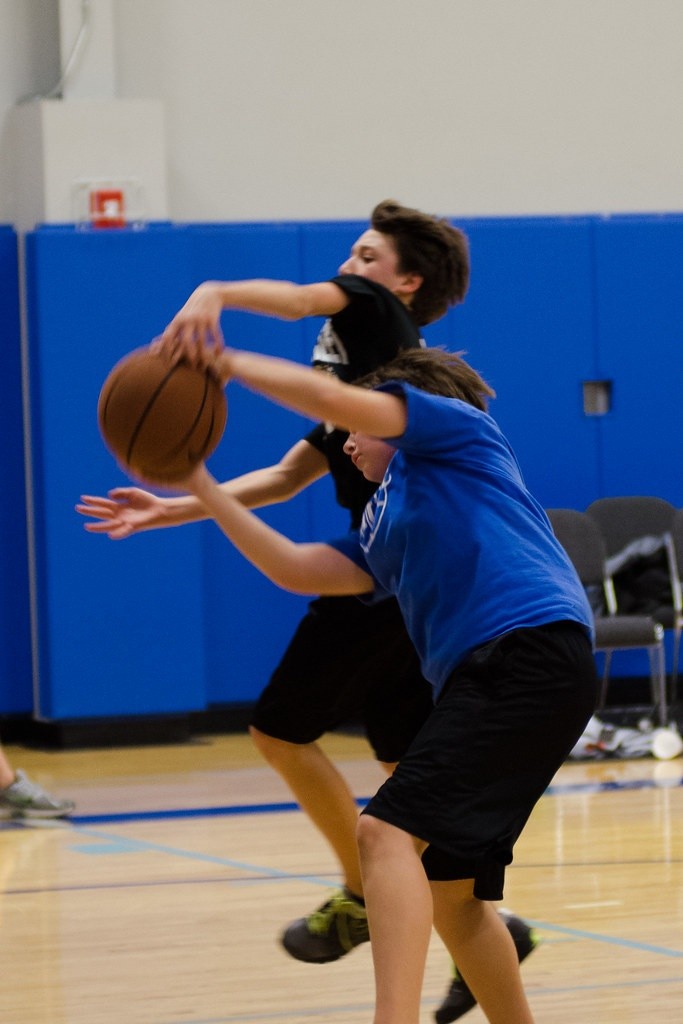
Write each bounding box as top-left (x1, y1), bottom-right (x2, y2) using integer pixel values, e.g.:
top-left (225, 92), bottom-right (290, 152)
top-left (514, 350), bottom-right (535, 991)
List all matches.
top-left (283, 886), bottom-right (370, 964)
top-left (0, 769), bottom-right (74, 819)
top-left (435, 909), bottom-right (540, 1024)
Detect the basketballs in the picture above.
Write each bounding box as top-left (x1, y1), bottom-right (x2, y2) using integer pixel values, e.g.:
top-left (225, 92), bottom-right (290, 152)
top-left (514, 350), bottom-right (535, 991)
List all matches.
top-left (96, 345), bottom-right (229, 482)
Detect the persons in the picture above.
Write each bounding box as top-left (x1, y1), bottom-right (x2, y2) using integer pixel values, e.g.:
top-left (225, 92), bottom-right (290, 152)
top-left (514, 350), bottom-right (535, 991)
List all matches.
top-left (77, 200), bottom-right (543, 1024)
top-left (0, 750), bottom-right (75, 818)
top-left (166, 342), bottom-right (604, 1024)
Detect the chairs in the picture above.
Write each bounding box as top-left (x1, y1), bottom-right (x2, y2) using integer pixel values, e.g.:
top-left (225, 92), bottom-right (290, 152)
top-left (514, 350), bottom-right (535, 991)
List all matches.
top-left (543, 508), bottom-right (666, 728)
top-left (583, 493), bottom-right (683, 725)
